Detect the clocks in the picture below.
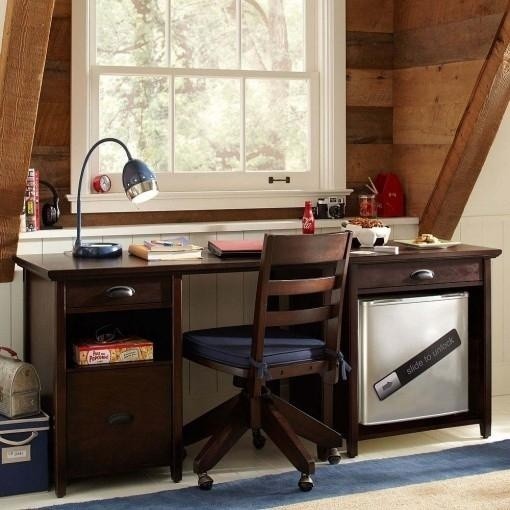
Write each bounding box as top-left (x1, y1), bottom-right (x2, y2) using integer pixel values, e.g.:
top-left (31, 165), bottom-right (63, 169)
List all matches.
top-left (92, 175), bottom-right (112, 194)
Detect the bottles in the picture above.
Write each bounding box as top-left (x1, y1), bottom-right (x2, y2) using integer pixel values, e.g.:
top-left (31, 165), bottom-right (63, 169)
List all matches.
top-left (301, 202), bottom-right (315, 233)
top-left (358, 194), bottom-right (379, 218)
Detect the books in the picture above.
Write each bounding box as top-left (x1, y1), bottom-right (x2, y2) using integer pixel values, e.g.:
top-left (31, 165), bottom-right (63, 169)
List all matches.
top-left (21, 167), bottom-right (39, 233)
top-left (128, 239), bottom-right (263, 262)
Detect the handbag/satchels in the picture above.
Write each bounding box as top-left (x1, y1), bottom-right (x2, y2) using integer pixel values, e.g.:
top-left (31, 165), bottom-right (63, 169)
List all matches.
top-left (0, 347), bottom-right (41, 420)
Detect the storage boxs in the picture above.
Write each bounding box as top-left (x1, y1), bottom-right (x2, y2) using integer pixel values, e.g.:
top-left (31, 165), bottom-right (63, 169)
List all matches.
top-left (0, 412), bottom-right (51, 495)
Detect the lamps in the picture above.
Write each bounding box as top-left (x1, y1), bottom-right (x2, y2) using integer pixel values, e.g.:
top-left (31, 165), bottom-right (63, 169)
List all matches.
top-left (71, 137), bottom-right (160, 259)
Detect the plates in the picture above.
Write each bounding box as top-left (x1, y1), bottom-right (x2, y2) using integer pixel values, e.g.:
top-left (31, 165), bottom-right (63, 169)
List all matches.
top-left (395, 238), bottom-right (460, 248)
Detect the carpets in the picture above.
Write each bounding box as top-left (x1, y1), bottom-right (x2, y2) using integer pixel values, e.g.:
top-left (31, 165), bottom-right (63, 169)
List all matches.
top-left (41, 437), bottom-right (509, 510)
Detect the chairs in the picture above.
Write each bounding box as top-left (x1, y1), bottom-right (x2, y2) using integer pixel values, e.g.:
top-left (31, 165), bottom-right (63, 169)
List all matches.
top-left (181, 231), bottom-right (354, 492)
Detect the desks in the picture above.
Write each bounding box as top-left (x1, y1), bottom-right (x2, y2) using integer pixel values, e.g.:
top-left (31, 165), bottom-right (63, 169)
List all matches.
top-left (14, 234), bottom-right (502, 498)
